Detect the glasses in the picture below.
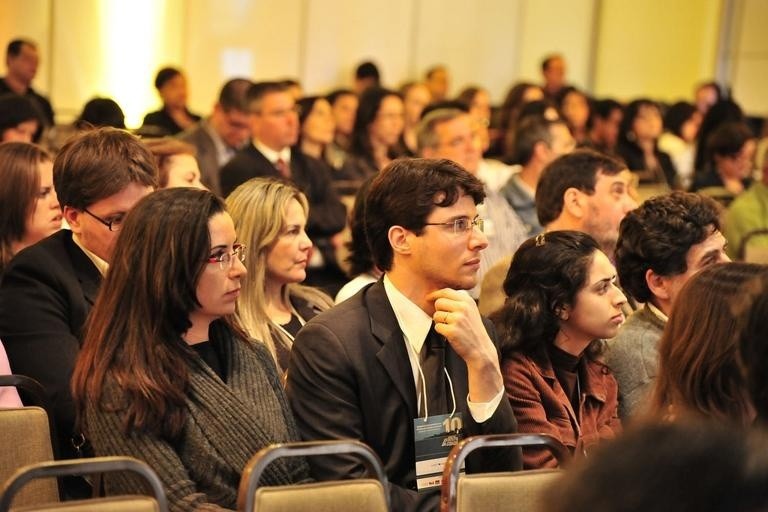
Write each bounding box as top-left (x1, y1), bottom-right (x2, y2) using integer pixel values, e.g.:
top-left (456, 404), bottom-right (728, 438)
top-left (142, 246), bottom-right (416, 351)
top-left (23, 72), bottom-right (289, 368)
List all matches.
top-left (78, 204), bottom-right (126, 234)
top-left (425, 216), bottom-right (486, 238)
top-left (199, 241), bottom-right (249, 271)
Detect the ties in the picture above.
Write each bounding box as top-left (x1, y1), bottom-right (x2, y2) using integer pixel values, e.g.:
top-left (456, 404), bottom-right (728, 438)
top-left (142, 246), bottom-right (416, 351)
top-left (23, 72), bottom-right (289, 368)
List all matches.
top-left (275, 158), bottom-right (292, 181)
top-left (418, 322), bottom-right (454, 420)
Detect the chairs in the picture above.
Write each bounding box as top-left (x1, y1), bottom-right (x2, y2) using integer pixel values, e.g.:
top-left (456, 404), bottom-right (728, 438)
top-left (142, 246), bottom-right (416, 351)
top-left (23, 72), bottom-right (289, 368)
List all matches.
top-left (620, 169), bottom-right (768, 271)
top-left (237, 439), bottom-right (397, 510)
top-left (0, 456), bottom-right (167, 512)
top-left (0, 371), bottom-right (68, 511)
top-left (438, 433), bottom-right (572, 511)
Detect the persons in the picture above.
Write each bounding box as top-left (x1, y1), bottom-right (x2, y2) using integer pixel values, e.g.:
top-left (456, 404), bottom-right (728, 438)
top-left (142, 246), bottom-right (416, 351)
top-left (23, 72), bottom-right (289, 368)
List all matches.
top-left (0, 40), bottom-right (54, 126)
